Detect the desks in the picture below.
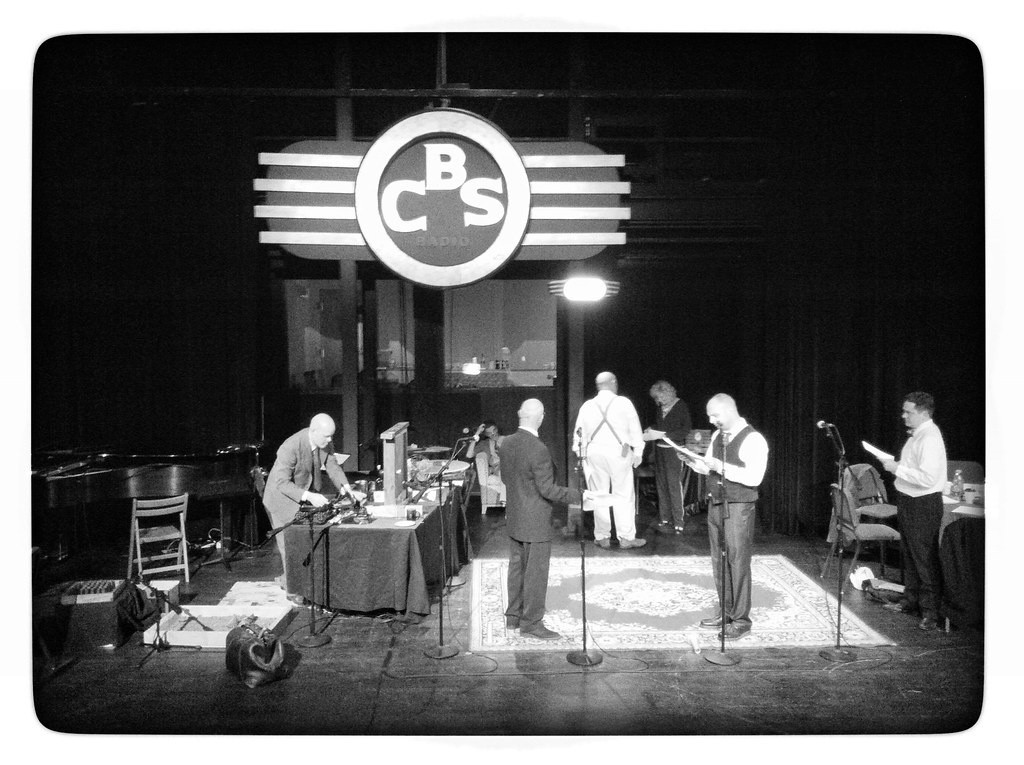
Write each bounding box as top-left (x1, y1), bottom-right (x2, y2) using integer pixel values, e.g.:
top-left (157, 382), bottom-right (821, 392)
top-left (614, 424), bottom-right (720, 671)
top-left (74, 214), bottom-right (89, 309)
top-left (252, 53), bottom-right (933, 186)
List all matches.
top-left (288, 475), bottom-right (472, 616)
top-left (943, 482), bottom-right (985, 630)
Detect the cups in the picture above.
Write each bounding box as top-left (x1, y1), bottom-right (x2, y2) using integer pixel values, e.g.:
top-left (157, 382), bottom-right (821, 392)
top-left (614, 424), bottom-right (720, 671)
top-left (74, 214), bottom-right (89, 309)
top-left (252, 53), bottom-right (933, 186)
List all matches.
top-left (407, 508), bottom-right (421, 521)
top-left (964, 490), bottom-right (976, 505)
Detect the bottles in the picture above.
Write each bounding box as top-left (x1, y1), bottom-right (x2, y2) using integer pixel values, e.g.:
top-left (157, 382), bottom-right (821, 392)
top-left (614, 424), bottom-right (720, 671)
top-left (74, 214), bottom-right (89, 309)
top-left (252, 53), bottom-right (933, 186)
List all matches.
top-left (949, 469), bottom-right (963, 501)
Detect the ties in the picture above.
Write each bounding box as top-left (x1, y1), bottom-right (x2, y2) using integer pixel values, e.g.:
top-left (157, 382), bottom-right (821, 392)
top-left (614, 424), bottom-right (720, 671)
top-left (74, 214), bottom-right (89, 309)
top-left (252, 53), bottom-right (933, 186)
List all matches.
top-left (313, 448), bottom-right (322, 492)
top-left (494, 441), bottom-right (500, 456)
top-left (723, 433), bottom-right (731, 446)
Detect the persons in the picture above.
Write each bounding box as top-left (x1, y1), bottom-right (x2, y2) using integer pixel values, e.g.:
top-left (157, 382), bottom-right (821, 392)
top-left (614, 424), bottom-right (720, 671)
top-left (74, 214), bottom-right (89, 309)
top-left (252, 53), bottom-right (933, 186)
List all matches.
top-left (677, 393), bottom-right (769, 641)
top-left (262, 413), bottom-right (356, 605)
top-left (571, 372), bottom-right (646, 549)
top-left (878, 392), bottom-right (947, 631)
top-left (499, 398), bottom-right (595, 641)
top-left (644, 381), bottom-right (692, 536)
top-left (466, 421), bottom-right (507, 519)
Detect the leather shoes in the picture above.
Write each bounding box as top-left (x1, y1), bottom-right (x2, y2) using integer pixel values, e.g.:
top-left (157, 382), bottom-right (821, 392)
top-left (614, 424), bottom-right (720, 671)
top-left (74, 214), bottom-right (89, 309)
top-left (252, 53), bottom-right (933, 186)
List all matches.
top-left (507, 622), bottom-right (520, 629)
top-left (520, 627), bottom-right (561, 640)
top-left (700, 616), bottom-right (731, 629)
top-left (718, 623), bottom-right (751, 641)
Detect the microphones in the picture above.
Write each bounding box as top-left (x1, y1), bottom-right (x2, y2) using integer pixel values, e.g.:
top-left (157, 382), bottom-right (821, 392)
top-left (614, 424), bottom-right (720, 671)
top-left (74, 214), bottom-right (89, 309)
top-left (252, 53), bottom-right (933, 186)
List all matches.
top-left (709, 416), bottom-right (722, 427)
top-left (816, 420), bottom-right (835, 428)
top-left (461, 434), bottom-right (481, 443)
top-left (340, 483), bottom-right (361, 510)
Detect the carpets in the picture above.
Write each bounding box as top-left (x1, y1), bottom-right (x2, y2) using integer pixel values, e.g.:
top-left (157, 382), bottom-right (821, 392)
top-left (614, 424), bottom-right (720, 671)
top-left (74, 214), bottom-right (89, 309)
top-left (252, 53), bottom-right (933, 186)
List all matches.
top-left (468, 553), bottom-right (893, 653)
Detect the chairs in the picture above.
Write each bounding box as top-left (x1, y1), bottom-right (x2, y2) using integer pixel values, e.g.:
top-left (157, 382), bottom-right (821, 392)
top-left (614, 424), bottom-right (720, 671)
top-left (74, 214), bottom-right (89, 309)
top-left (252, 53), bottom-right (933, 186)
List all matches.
top-left (127, 493), bottom-right (192, 585)
top-left (826, 461), bottom-right (985, 604)
top-left (478, 453), bottom-right (506, 514)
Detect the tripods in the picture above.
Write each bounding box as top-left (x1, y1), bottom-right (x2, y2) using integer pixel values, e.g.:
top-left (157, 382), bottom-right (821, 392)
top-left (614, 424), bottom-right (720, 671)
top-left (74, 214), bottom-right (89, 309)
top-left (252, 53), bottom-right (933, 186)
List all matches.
top-left (201, 499), bottom-right (241, 574)
top-left (135, 579), bottom-right (203, 669)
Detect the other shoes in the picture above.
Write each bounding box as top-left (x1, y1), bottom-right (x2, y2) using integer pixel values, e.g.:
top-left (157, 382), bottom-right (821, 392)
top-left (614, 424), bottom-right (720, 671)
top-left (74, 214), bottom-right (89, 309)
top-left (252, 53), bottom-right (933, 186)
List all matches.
top-left (882, 603), bottom-right (903, 612)
top-left (655, 521), bottom-right (667, 528)
top-left (619, 538), bottom-right (646, 549)
top-left (674, 526), bottom-right (683, 535)
top-left (286, 594), bottom-right (304, 607)
top-left (918, 618), bottom-right (939, 630)
top-left (594, 538), bottom-right (610, 549)
top-left (500, 500), bottom-right (506, 507)
